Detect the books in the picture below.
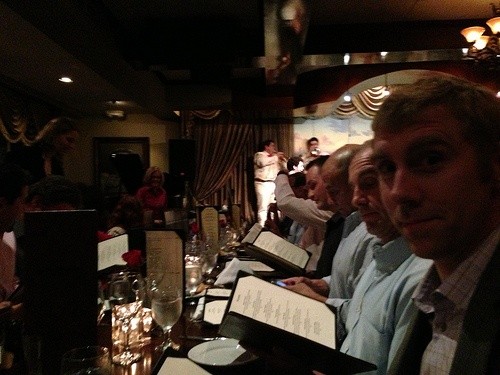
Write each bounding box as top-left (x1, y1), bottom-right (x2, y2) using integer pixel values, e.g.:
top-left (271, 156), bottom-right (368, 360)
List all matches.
top-left (217, 270), bottom-right (378, 375)
top-left (240, 221), bottom-right (268, 244)
top-left (243, 228), bottom-right (312, 275)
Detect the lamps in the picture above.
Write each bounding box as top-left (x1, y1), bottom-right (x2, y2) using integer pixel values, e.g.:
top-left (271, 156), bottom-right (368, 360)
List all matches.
top-left (460, 3), bottom-right (500, 72)
top-left (104, 109), bottom-right (127, 122)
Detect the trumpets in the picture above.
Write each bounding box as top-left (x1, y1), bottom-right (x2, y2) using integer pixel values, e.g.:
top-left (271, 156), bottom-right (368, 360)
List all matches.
top-left (270, 150), bottom-right (287, 163)
top-left (310, 146), bottom-right (321, 155)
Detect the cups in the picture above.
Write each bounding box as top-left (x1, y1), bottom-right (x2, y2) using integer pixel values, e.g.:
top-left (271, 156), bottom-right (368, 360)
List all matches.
top-left (232, 228), bottom-right (240, 244)
top-left (184, 240), bottom-right (206, 263)
top-left (62, 345), bottom-right (113, 375)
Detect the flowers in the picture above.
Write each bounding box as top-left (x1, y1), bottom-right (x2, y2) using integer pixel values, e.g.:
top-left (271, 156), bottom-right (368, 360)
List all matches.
top-left (218, 220), bottom-right (227, 229)
top-left (122, 250), bottom-right (146, 272)
top-left (189, 222), bottom-right (200, 236)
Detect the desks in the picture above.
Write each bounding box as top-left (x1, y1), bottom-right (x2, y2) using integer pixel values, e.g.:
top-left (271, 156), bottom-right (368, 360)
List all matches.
top-left (96, 221), bottom-right (293, 375)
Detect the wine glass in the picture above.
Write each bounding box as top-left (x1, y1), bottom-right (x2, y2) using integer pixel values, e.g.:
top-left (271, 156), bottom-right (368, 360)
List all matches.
top-left (151, 296), bottom-right (182, 353)
top-left (108, 273), bottom-right (145, 364)
top-left (185, 265), bottom-right (202, 297)
top-left (219, 228), bottom-right (234, 255)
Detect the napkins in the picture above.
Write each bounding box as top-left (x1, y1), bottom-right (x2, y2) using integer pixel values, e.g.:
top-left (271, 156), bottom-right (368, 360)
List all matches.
top-left (214, 257), bottom-right (253, 286)
top-left (192, 296), bottom-right (205, 320)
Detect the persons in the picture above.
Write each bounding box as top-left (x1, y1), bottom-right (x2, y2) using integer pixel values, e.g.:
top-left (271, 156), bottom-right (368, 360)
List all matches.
top-left (273, 76), bottom-right (500, 374)
top-left (135, 166), bottom-right (167, 212)
top-left (254, 140), bottom-right (287, 223)
top-left (0, 116), bottom-right (143, 301)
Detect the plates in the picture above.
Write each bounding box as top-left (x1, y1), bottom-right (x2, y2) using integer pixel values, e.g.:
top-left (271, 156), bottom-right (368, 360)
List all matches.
top-left (188, 338), bottom-right (258, 367)
